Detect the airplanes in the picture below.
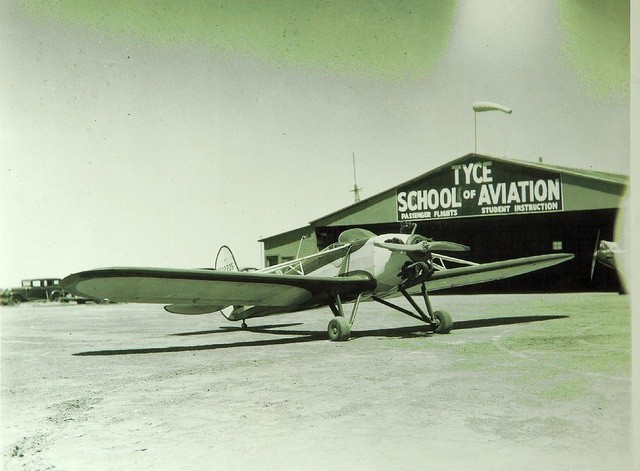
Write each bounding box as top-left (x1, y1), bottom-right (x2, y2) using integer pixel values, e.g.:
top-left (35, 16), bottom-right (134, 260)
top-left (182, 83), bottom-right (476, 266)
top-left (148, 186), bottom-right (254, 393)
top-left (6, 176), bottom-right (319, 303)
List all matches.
top-left (59, 221), bottom-right (576, 341)
top-left (590, 229), bottom-right (631, 281)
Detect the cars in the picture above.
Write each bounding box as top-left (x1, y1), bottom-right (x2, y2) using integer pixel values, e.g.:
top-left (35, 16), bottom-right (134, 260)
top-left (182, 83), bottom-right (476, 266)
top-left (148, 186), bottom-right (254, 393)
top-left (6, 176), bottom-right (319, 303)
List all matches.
top-left (0, 278), bottom-right (66, 305)
